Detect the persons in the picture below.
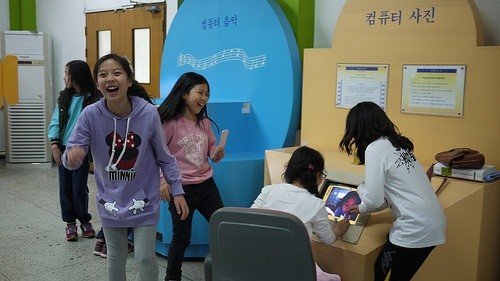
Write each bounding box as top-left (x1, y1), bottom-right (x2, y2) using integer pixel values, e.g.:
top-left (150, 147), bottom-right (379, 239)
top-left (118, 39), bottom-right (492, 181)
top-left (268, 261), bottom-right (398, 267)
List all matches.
top-left (339, 102), bottom-right (447, 280)
top-left (93, 55), bottom-right (134, 258)
top-left (61, 53), bottom-right (189, 281)
top-left (156, 72), bottom-right (229, 281)
top-left (47, 60), bottom-right (103, 241)
top-left (250, 146), bottom-right (350, 281)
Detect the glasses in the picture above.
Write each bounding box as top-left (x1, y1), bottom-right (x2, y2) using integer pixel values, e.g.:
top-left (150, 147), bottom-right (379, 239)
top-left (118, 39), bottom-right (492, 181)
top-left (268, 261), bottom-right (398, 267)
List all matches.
top-left (320, 171), bottom-right (327, 180)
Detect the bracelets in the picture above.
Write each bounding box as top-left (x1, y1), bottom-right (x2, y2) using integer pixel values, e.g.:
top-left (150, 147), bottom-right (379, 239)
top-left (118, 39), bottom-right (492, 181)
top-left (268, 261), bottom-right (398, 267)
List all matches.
top-left (50, 145), bottom-right (58, 149)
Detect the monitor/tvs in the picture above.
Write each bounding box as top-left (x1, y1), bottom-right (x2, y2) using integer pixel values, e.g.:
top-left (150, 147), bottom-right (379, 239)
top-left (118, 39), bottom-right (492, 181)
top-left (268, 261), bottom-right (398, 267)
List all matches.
top-left (317, 181), bottom-right (363, 226)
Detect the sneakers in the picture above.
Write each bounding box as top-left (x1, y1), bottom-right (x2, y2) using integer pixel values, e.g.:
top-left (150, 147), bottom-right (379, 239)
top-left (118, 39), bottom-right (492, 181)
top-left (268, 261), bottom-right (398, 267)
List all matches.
top-left (93, 240), bottom-right (133, 258)
top-left (65, 222), bottom-right (96, 242)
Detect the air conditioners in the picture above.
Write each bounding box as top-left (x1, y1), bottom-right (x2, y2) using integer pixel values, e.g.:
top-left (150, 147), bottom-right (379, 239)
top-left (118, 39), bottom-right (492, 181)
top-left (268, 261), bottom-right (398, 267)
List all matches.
top-left (1, 30), bottom-right (55, 169)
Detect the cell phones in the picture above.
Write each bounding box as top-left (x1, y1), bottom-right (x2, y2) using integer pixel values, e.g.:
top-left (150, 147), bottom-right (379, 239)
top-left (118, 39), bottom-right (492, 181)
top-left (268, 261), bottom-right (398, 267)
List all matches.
top-left (211, 130), bottom-right (229, 160)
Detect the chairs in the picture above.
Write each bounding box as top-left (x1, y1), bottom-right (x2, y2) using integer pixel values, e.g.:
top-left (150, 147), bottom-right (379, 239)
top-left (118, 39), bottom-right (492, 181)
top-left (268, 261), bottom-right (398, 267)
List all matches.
top-left (204, 207), bottom-right (323, 281)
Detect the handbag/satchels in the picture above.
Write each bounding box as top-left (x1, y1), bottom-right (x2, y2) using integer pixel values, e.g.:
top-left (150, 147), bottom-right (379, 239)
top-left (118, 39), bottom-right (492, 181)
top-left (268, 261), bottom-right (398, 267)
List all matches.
top-left (426, 147), bottom-right (485, 195)
